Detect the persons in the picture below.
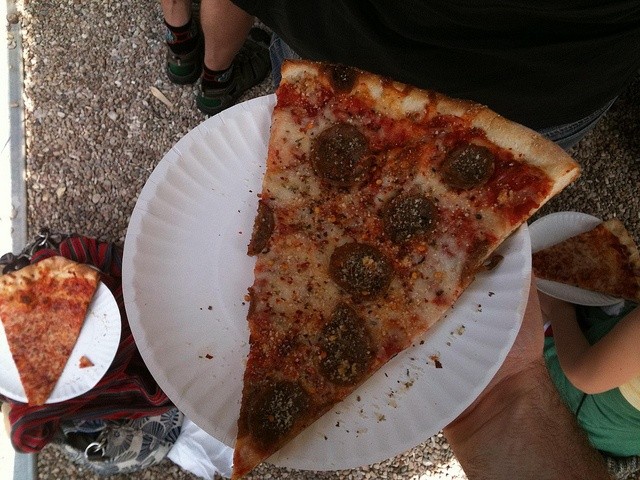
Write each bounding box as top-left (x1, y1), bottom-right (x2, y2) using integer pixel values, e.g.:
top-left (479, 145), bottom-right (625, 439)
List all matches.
top-left (159, 0), bottom-right (272, 114)
top-left (539, 278), bottom-right (639, 458)
top-left (232, 0), bottom-right (640, 152)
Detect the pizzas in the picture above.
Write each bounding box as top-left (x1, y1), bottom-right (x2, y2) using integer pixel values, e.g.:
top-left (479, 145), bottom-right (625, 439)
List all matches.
top-left (532, 219), bottom-right (640, 310)
top-left (1, 257), bottom-right (101, 409)
top-left (228, 57), bottom-right (579, 476)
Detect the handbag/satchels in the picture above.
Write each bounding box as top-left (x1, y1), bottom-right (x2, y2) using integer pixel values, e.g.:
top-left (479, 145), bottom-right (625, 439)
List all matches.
top-left (0, 225), bottom-right (183, 475)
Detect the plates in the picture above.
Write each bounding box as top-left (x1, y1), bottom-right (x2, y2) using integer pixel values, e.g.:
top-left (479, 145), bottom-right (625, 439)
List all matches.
top-left (122, 95), bottom-right (532, 471)
top-left (0, 280), bottom-right (122, 405)
top-left (530, 211), bottom-right (627, 315)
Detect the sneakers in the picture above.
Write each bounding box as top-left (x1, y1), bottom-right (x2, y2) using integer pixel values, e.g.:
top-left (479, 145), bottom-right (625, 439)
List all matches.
top-left (196, 27), bottom-right (272, 115)
top-left (164, 39), bottom-right (202, 85)
